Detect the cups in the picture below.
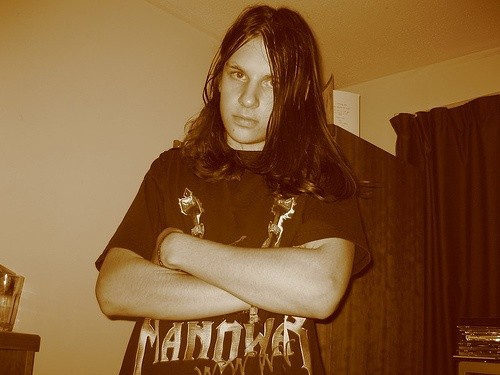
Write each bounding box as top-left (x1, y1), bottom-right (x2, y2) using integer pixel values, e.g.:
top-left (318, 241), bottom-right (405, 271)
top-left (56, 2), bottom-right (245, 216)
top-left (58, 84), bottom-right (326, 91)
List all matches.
top-left (0, 273), bottom-right (25, 331)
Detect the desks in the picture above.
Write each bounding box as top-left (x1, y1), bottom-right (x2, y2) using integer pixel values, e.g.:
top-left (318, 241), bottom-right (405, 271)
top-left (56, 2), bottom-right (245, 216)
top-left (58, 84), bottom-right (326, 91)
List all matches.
top-left (0, 332), bottom-right (41, 375)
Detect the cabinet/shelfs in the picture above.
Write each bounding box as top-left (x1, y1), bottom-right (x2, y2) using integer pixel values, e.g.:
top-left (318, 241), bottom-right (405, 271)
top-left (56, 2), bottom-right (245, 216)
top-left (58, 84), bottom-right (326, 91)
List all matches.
top-left (317, 125), bottom-right (395, 374)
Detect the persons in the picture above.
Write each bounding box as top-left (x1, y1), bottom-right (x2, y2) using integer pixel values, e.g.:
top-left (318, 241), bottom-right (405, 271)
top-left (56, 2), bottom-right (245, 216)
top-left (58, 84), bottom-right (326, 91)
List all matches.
top-left (93, 4), bottom-right (373, 375)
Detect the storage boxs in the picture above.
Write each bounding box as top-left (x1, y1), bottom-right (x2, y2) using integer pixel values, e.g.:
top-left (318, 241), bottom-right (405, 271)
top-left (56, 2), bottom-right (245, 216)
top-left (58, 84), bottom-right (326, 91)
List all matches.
top-left (321, 74), bottom-right (362, 137)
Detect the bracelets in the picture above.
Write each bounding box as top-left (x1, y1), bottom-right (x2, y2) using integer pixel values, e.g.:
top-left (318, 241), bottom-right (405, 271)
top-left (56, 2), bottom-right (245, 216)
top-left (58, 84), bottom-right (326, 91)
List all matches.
top-left (156, 229), bottom-right (186, 271)
